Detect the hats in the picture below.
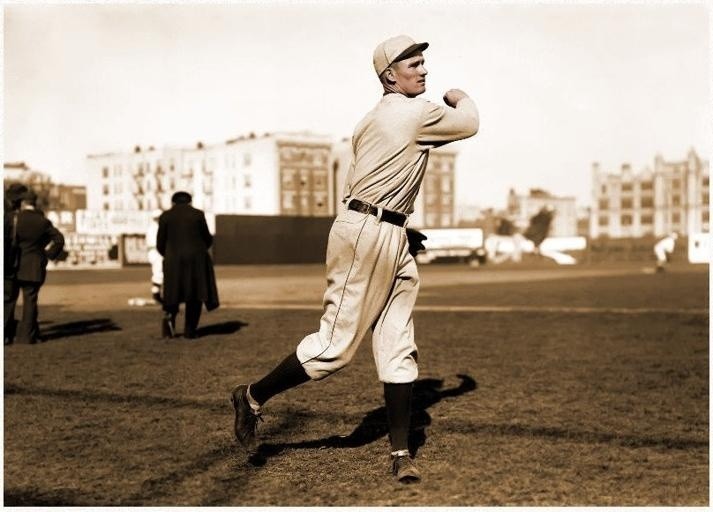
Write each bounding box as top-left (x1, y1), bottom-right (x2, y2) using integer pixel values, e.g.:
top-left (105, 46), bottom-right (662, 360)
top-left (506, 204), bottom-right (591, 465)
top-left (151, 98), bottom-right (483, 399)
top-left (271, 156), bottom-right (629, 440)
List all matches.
top-left (372, 34), bottom-right (429, 76)
top-left (172, 191), bottom-right (192, 204)
top-left (19, 193), bottom-right (36, 201)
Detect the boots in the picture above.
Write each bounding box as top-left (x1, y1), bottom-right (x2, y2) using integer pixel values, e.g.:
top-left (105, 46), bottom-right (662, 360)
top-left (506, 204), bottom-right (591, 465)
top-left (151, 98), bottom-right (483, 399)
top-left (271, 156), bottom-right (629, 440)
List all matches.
top-left (160, 315), bottom-right (176, 338)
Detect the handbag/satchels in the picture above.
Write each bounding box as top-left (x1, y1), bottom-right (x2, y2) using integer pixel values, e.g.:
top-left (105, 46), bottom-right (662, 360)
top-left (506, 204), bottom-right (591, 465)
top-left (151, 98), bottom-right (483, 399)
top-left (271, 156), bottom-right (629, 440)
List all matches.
top-left (4, 244), bottom-right (19, 274)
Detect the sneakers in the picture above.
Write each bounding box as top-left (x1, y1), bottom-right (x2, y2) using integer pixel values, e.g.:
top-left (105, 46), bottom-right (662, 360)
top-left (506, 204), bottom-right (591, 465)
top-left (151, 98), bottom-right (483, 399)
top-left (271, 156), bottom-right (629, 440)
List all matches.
top-left (392, 456), bottom-right (420, 483)
top-left (231, 385), bottom-right (257, 446)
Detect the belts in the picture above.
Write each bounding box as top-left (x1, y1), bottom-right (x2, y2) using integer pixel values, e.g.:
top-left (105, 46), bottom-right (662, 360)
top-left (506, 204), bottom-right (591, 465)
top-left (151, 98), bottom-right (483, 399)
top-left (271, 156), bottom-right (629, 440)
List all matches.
top-left (344, 198), bottom-right (410, 227)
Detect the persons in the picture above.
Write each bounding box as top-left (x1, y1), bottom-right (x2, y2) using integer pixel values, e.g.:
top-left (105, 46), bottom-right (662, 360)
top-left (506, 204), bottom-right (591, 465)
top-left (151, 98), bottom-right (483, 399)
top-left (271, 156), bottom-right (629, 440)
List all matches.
top-left (147, 191), bottom-right (219, 339)
top-left (653, 232), bottom-right (678, 270)
top-left (232, 35), bottom-right (479, 481)
top-left (4, 192), bottom-right (65, 346)
